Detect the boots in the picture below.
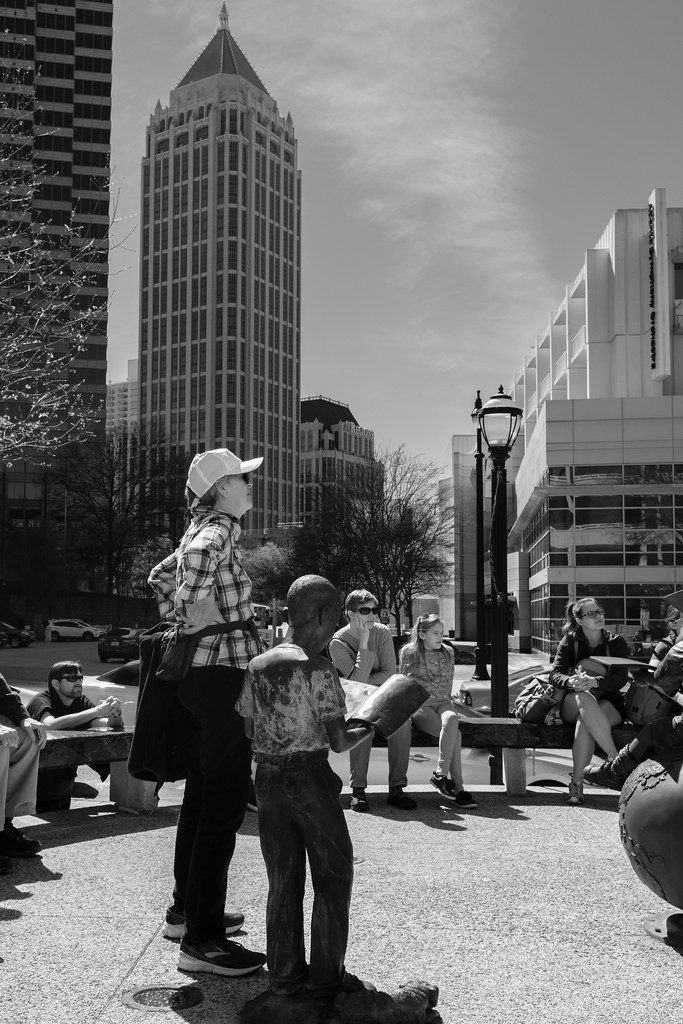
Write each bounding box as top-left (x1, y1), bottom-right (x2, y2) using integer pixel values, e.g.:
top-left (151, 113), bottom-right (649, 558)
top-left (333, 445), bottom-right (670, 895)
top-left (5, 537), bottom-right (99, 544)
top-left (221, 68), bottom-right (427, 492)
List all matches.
top-left (582, 744), bottom-right (636, 792)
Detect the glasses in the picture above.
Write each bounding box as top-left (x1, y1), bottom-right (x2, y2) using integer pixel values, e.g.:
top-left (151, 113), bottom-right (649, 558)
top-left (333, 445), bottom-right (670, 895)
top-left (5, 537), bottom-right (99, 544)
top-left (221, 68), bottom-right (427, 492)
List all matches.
top-left (62, 674), bottom-right (84, 682)
top-left (359, 607), bottom-right (379, 615)
top-left (579, 609), bottom-right (605, 619)
top-left (234, 473), bottom-right (249, 484)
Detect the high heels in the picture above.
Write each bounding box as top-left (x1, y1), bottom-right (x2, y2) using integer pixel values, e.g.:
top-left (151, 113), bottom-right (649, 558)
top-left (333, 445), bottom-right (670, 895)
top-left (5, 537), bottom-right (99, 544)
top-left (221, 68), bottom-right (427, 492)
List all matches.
top-left (568, 779), bottom-right (585, 806)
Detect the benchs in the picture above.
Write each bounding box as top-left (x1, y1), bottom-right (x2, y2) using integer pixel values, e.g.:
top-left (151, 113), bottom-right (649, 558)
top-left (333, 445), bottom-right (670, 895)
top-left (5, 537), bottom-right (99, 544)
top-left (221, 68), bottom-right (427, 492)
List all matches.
top-left (39, 717), bottom-right (641, 815)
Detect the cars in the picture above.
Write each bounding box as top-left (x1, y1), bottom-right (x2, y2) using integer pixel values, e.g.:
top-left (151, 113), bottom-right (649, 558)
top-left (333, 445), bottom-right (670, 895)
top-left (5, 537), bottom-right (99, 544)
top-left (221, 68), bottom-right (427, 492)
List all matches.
top-left (98, 627), bottom-right (148, 663)
top-left (460, 663), bottom-right (551, 714)
top-left (10, 660), bottom-right (141, 729)
top-left (0, 631), bottom-right (8, 647)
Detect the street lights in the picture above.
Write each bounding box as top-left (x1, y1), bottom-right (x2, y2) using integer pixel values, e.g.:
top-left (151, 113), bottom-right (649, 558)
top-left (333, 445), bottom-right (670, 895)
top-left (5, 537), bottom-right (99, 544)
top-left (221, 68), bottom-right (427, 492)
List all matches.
top-left (472, 397), bottom-right (490, 680)
top-left (480, 387), bottom-right (524, 785)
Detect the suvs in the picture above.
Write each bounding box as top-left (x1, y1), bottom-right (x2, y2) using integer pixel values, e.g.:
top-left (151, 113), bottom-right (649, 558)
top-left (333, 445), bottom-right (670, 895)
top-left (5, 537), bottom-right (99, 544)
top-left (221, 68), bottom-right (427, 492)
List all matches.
top-left (45, 618), bottom-right (104, 642)
top-left (0, 622), bottom-right (37, 648)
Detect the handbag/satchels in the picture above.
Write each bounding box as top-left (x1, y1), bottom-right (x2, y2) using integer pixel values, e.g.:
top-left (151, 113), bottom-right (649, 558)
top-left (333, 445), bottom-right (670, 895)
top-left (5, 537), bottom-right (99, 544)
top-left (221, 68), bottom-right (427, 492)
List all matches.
top-left (124, 619), bottom-right (198, 780)
top-left (514, 674), bottom-right (564, 722)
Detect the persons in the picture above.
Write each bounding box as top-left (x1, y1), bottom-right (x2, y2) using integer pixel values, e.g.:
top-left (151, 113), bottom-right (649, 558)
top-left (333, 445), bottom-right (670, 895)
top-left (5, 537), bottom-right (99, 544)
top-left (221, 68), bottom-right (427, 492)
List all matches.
top-left (236, 574), bottom-right (376, 1000)
top-left (549, 598), bottom-right (631, 803)
top-left (582, 642), bottom-right (683, 791)
top-left (148, 447), bottom-right (266, 975)
top-left (329, 589), bottom-right (418, 812)
top-left (649, 605), bottom-right (683, 673)
top-left (399, 614), bottom-right (477, 809)
top-left (0, 674), bottom-right (48, 875)
top-left (28, 661), bottom-right (123, 812)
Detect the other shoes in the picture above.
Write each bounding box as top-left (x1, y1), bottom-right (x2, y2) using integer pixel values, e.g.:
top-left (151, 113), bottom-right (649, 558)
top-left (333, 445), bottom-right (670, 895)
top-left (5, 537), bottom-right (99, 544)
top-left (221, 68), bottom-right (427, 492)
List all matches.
top-left (0, 821), bottom-right (42, 858)
top-left (0, 859), bottom-right (11, 873)
top-left (247, 787), bottom-right (258, 813)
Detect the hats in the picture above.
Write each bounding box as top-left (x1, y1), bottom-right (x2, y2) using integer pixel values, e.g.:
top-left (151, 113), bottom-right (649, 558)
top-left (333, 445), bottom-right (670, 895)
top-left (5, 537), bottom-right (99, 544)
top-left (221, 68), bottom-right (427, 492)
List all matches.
top-left (186, 448), bottom-right (263, 507)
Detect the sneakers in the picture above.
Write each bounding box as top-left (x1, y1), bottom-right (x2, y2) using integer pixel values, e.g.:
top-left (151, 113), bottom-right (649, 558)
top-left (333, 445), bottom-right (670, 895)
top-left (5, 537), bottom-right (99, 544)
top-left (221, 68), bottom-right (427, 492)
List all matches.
top-left (161, 901), bottom-right (244, 938)
top-left (451, 790), bottom-right (478, 808)
top-left (269, 962), bottom-right (312, 996)
top-left (351, 790), bottom-right (369, 812)
top-left (387, 788), bottom-right (418, 809)
top-left (430, 772), bottom-right (457, 801)
top-left (177, 922), bottom-right (266, 975)
top-left (340, 969), bottom-right (376, 995)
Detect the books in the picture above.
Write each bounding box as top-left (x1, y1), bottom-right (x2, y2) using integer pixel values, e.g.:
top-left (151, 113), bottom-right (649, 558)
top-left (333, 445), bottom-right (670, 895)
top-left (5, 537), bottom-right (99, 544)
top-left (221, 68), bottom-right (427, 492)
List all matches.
top-left (343, 673), bottom-right (430, 739)
